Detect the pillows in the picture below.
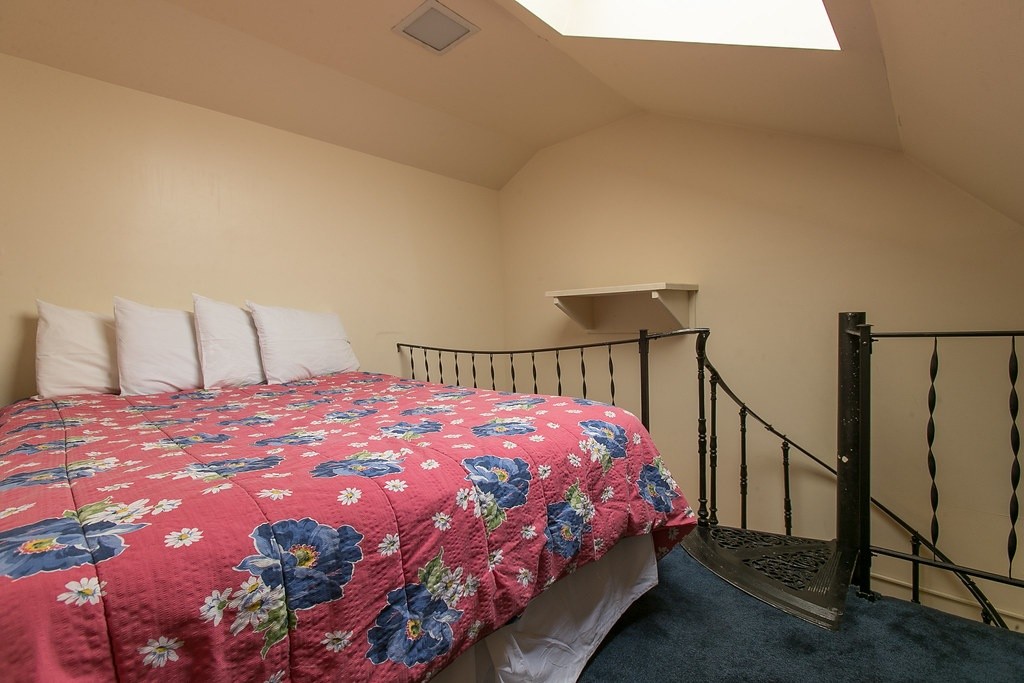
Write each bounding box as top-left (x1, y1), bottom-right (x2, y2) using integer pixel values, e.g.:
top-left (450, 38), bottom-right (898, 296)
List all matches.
top-left (244, 299), bottom-right (360, 386)
top-left (110, 296), bottom-right (204, 397)
top-left (29, 298), bottom-right (120, 402)
top-left (190, 291), bottom-right (268, 391)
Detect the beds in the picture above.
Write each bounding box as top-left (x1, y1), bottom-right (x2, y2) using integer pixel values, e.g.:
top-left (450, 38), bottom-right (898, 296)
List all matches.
top-left (1, 368), bottom-right (697, 683)
